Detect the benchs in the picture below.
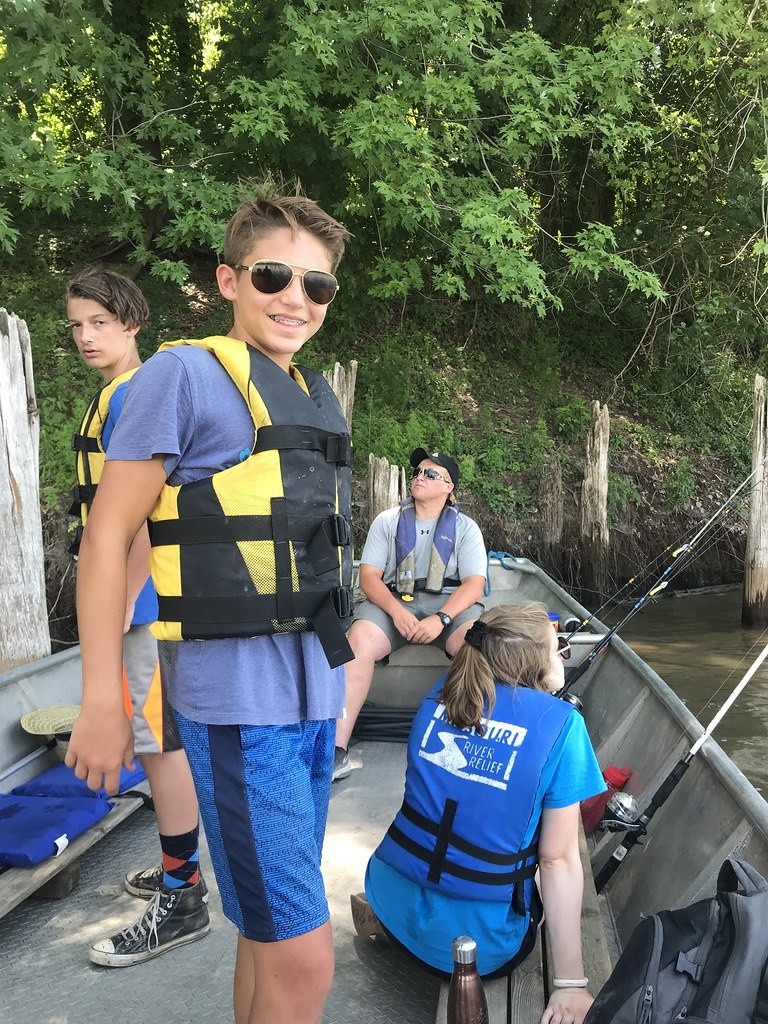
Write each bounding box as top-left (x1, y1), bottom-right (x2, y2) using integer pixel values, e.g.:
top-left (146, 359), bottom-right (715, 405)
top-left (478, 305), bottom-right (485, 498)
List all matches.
top-left (0, 794), bottom-right (145, 921)
top-left (436, 811), bottom-right (612, 1024)
top-left (11, 758), bottom-right (154, 814)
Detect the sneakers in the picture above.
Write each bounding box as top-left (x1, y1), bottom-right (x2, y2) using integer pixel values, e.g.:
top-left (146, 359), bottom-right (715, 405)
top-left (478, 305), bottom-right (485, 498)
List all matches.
top-left (124, 859), bottom-right (208, 903)
top-left (89, 879), bottom-right (211, 968)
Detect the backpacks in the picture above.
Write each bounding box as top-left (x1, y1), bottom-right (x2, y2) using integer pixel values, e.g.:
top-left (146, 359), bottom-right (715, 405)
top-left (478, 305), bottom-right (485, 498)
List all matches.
top-left (584, 858), bottom-right (768, 1024)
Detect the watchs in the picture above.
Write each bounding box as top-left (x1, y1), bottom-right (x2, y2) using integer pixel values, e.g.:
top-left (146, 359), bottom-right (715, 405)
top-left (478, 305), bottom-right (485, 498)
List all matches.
top-left (434, 611), bottom-right (452, 629)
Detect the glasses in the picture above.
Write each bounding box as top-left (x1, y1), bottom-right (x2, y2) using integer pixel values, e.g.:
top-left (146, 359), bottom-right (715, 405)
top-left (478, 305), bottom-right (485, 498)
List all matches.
top-left (548, 636), bottom-right (572, 662)
top-left (232, 259), bottom-right (341, 307)
top-left (412, 468), bottom-right (451, 485)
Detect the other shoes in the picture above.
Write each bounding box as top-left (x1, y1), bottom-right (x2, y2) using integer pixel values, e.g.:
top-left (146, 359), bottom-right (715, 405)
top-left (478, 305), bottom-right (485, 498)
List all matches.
top-left (331, 745), bottom-right (352, 781)
top-left (351, 891), bottom-right (387, 940)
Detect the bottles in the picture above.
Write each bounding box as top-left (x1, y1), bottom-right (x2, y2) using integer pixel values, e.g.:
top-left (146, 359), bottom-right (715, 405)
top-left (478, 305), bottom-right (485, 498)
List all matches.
top-left (580, 765), bottom-right (633, 839)
top-left (548, 612), bottom-right (560, 633)
top-left (447, 935), bottom-right (488, 1024)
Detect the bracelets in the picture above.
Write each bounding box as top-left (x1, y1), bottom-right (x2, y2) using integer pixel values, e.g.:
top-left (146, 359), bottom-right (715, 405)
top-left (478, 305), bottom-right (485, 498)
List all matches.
top-left (552, 975), bottom-right (591, 990)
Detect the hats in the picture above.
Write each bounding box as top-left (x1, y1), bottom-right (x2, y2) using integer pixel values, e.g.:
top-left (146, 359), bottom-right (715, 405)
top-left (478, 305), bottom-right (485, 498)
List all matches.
top-left (410, 447), bottom-right (459, 489)
top-left (21, 705), bottom-right (81, 756)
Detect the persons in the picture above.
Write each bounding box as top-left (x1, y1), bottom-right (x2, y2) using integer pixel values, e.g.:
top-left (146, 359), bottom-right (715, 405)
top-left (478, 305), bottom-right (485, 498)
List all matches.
top-left (331, 446), bottom-right (488, 782)
top-left (61, 267), bottom-right (221, 970)
top-left (64, 192), bottom-right (357, 1022)
top-left (347, 599), bottom-right (601, 1024)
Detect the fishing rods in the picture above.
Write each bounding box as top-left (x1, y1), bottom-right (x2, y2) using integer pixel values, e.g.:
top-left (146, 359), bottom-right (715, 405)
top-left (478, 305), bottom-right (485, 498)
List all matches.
top-left (592, 627), bottom-right (767, 897)
top-left (550, 452), bottom-right (768, 705)
